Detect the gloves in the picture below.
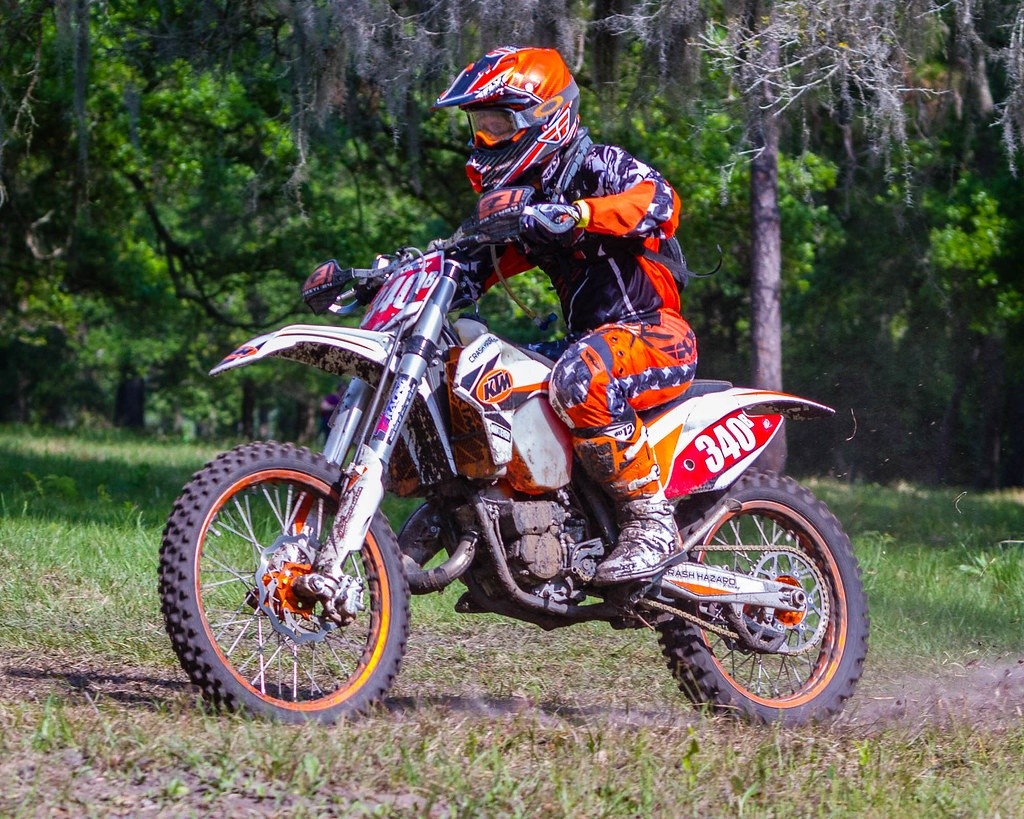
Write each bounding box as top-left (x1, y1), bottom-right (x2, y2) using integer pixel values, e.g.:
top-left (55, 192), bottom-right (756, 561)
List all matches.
top-left (369, 254), bottom-right (394, 289)
top-left (516, 200), bottom-right (581, 242)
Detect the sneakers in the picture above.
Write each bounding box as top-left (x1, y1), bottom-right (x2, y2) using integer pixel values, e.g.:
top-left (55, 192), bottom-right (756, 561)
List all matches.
top-left (596, 499), bottom-right (687, 582)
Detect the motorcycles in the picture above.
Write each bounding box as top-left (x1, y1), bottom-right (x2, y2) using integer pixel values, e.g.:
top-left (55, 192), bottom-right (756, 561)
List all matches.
top-left (156, 189), bottom-right (873, 732)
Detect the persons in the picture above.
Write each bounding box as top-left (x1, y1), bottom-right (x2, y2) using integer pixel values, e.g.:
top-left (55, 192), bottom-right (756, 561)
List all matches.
top-left (354, 46), bottom-right (696, 613)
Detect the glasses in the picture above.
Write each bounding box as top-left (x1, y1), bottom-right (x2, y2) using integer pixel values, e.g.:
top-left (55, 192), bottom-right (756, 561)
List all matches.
top-left (466, 106), bottom-right (523, 148)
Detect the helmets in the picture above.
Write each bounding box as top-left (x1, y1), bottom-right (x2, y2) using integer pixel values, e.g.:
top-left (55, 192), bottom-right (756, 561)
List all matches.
top-left (431, 46), bottom-right (582, 192)
